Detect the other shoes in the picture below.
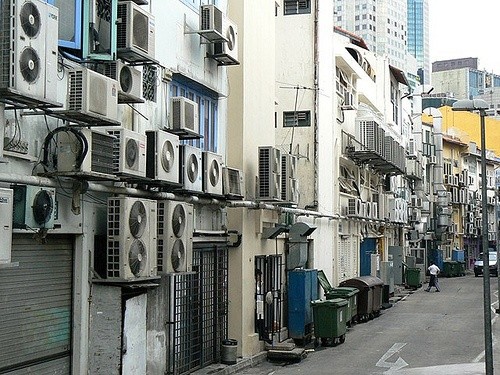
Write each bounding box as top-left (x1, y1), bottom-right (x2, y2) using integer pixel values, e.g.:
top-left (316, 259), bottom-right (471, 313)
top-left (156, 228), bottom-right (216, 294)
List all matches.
top-left (424, 290), bottom-right (429, 292)
top-left (435, 290), bottom-right (440, 292)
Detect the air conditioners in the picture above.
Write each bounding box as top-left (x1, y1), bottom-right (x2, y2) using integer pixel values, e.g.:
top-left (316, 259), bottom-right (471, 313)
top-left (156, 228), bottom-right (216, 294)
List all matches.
top-left (179, 145), bottom-right (202, 190)
top-left (145, 129), bottom-right (180, 183)
top-left (106, 196), bottom-right (158, 279)
top-left (213, 15), bottom-right (239, 65)
top-left (171, 95), bottom-right (200, 139)
top-left (348, 198), bottom-right (423, 225)
top-left (257, 145), bottom-right (282, 201)
top-left (280, 154), bottom-right (298, 204)
top-left (102, 60), bottom-right (143, 103)
top-left (445, 174), bottom-right (495, 241)
top-left (117, 0), bottom-right (155, 62)
top-left (157, 200), bottom-right (195, 273)
top-left (345, 92), bottom-right (354, 109)
top-left (0, 0), bottom-right (60, 102)
top-left (355, 120), bottom-right (424, 193)
top-left (11, 184), bottom-right (56, 229)
top-left (66, 68), bottom-right (118, 121)
top-left (201, 151), bottom-right (224, 195)
top-left (429, 156), bottom-right (437, 164)
top-left (57, 129), bottom-right (116, 176)
top-left (222, 166), bottom-right (241, 196)
top-left (198, 4), bottom-right (223, 41)
top-left (93, 127), bottom-right (148, 177)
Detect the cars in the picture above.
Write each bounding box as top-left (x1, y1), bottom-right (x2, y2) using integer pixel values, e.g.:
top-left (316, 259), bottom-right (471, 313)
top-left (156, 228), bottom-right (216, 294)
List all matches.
top-left (473, 251), bottom-right (499, 277)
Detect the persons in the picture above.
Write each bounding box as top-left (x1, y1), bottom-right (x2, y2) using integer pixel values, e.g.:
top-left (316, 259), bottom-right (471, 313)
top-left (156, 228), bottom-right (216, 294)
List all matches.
top-left (424, 261), bottom-right (441, 292)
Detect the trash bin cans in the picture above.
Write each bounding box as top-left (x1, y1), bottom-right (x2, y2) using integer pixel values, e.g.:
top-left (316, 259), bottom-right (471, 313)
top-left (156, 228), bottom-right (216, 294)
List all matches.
top-left (457, 260), bottom-right (466, 277)
top-left (339, 274), bottom-right (384, 322)
top-left (444, 261), bottom-right (457, 276)
top-left (326, 288), bottom-right (360, 325)
top-left (309, 298), bottom-right (351, 345)
top-left (405, 267), bottom-right (424, 288)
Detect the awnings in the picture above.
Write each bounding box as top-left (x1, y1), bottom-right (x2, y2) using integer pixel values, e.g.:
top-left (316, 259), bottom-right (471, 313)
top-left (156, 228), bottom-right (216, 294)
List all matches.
top-left (390, 65), bottom-right (409, 86)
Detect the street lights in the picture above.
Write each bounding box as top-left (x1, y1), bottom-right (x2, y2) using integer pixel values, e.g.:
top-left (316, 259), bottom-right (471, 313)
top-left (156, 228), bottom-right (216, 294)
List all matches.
top-left (451, 98), bottom-right (494, 375)
